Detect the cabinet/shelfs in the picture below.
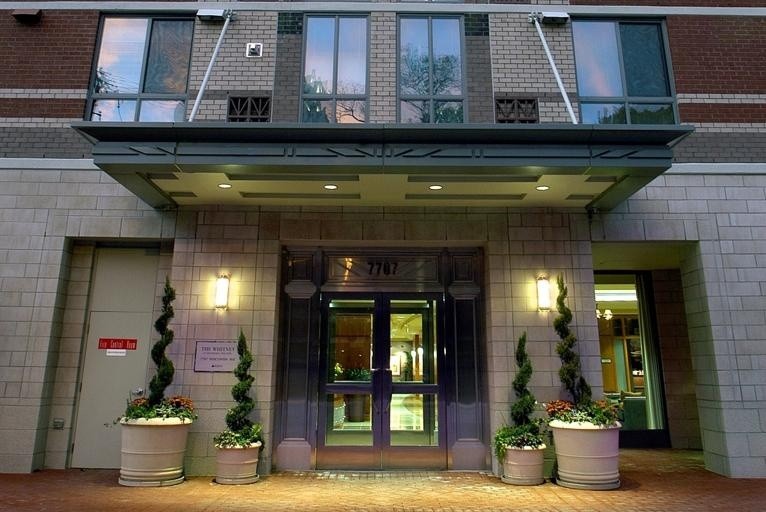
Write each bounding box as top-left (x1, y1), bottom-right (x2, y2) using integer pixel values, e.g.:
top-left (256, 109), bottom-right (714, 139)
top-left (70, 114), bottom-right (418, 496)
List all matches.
top-left (335, 314), bottom-right (371, 420)
top-left (597, 312), bottom-right (645, 396)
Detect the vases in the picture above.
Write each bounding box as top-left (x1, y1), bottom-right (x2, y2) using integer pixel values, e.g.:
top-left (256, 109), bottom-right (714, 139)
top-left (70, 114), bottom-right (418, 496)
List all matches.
top-left (334, 402), bottom-right (346, 429)
top-left (117, 414), bottom-right (185, 486)
top-left (549, 415), bottom-right (622, 490)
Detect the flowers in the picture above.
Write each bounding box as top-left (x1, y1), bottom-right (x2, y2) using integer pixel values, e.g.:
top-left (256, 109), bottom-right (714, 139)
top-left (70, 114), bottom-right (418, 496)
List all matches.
top-left (334, 393), bottom-right (344, 407)
top-left (105, 389), bottom-right (200, 430)
top-left (335, 362), bottom-right (344, 378)
top-left (543, 393), bottom-right (627, 428)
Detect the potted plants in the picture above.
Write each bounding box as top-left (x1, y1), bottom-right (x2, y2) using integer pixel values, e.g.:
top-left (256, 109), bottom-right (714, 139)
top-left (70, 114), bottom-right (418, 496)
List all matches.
top-left (213, 328), bottom-right (264, 485)
top-left (343, 368), bottom-right (372, 422)
top-left (491, 332), bottom-right (548, 487)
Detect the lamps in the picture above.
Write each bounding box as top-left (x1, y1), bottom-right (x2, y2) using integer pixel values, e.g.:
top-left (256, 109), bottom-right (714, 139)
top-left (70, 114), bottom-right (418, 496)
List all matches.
top-left (186, 7), bottom-right (239, 121)
top-left (536, 272), bottom-right (551, 314)
top-left (211, 272), bottom-right (231, 312)
top-left (529, 9), bottom-right (580, 124)
